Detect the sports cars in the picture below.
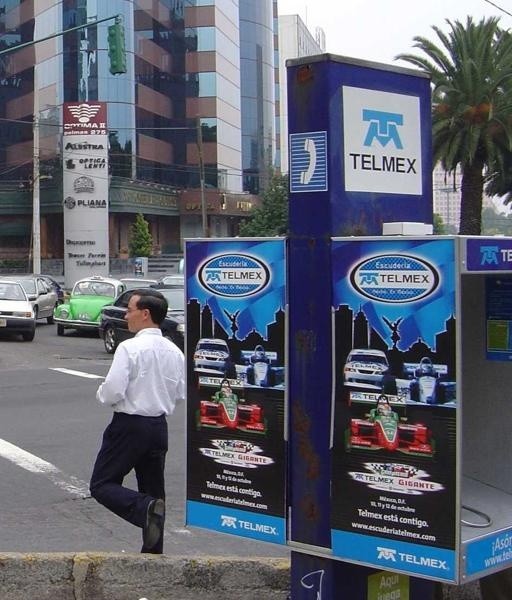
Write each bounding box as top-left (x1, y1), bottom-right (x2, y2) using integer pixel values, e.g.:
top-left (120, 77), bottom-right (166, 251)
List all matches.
top-left (195, 390), bottom-right (269, 440)
top-left (346, 403), bottom-right (438, 465)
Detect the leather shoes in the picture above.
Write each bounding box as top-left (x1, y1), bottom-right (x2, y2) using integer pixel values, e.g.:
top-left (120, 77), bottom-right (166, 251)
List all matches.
top-left (142, 498), bottom-right (166, 550)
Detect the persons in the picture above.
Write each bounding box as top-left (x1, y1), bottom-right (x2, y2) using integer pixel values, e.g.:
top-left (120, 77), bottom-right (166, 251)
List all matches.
top-left (89, 288), bottom-right (184, 554)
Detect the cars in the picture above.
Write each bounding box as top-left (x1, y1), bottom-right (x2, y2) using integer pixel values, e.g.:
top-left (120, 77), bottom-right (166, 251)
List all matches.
top-left (0, 273), bottom-right (184, 356)
top-left (343, 346), bottom-right (391, 391)
top-left (194, 337), bottom-right (229, 374)
